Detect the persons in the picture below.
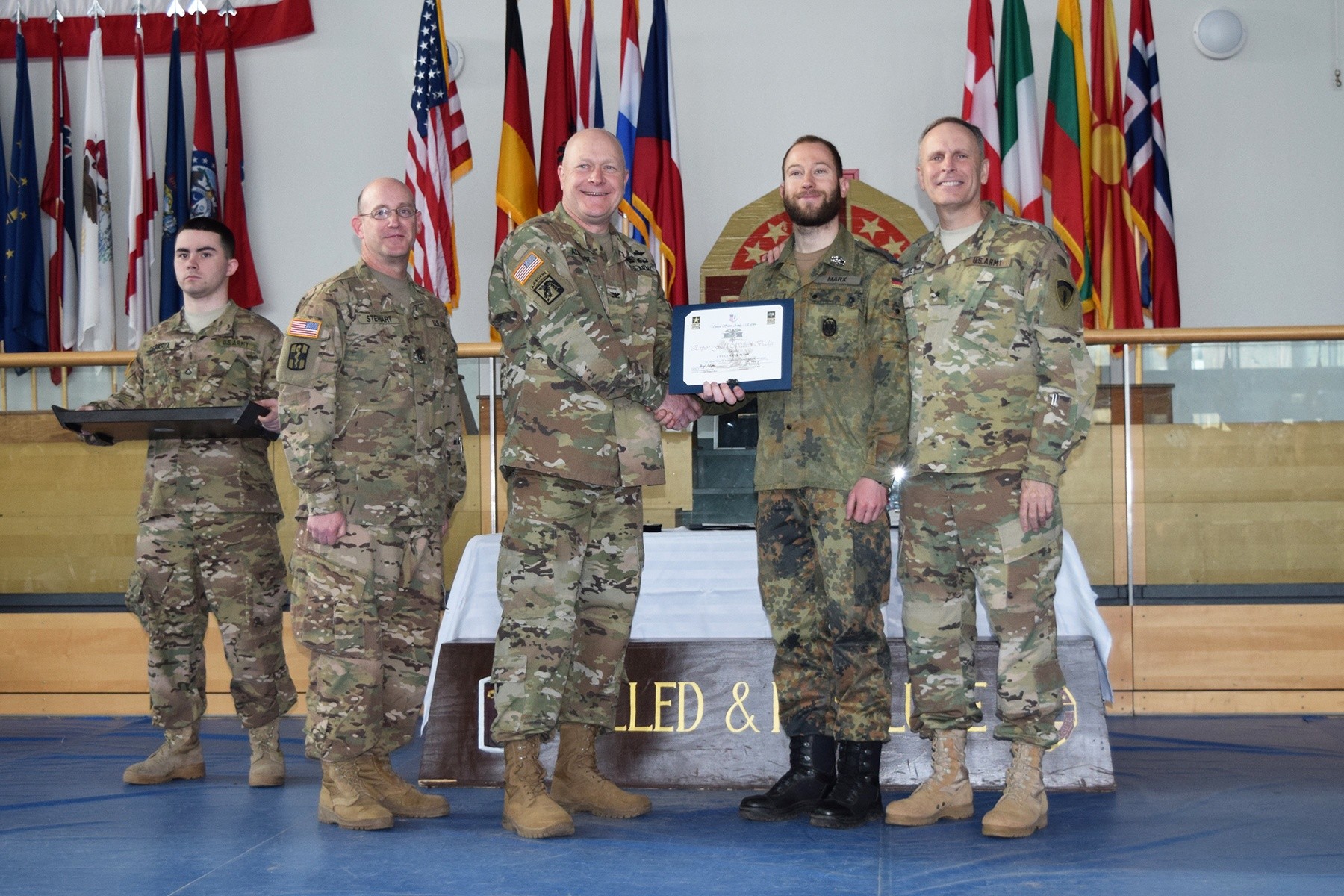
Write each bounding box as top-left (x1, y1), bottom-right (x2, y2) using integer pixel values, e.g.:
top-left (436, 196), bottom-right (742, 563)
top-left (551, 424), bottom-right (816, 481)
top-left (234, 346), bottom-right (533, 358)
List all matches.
top-left (488, 117), bottom-right (1096, 838)
top-left (77, 218), bottom-right (284, 789)
top-left (277, 178), bottom-right (465, 827)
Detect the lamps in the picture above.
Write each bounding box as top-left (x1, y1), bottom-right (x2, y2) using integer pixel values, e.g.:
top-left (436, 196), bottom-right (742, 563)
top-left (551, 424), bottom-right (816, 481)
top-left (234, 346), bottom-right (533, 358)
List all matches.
top-left (1193, 7), bottom-right (1247, 59)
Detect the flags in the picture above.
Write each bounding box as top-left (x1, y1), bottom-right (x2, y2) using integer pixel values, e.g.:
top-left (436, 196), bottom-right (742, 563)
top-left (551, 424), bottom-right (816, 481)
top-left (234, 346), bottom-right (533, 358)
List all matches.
top-left (0, 25), bottom-right (264, 384)
top-left (963, 0), bottom-right (1181, 329)
top-left (404, 0), bottom-right (688, 315)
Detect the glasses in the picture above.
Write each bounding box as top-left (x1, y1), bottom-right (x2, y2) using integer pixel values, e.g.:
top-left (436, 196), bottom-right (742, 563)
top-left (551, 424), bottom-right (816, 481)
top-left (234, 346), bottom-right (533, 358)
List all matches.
top-left (359, 206), bottom-right (418, 220)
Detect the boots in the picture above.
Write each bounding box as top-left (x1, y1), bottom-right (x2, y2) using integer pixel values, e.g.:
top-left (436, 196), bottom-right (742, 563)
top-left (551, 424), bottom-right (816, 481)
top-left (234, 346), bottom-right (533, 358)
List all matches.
top-left (550, 723), bottom-right (651, 818)
top-left (981, 740), bottom-right (1048, 837)
top-left (123, 718), bottom-right (206, 784)
top-left (740, 735), bottom-right (838, 820)
top-left (810, 741), bottom-right (882, 828)
top-left (248, 716), bottom-right (286, 787)
top-left (352, 754), bottom-right (450, 818)
top-left (318, 758), bottom-right (394, 829)
top-left (502, 736), bottom-right (575, 837)
top-left (885, 729), bottom-right (974, 826)
